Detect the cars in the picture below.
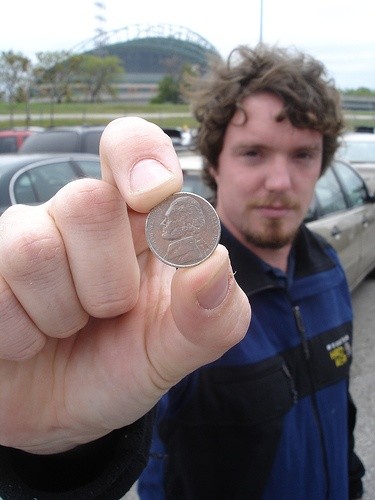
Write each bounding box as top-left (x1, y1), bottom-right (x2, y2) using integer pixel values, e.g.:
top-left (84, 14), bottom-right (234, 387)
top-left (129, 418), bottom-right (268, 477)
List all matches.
top-left (0, 155), bottom-right (106, 212)
top-left (334, 132), bottom-right (375, 199)
top-left (0, 129), bottom-right (32, 156)
top-left (177, 154), bottom-right (375, 296)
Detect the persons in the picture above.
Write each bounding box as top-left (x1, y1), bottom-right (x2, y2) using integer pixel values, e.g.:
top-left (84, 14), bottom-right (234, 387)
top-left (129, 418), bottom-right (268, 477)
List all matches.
top-left (0, 42), bottom-right (366, 500)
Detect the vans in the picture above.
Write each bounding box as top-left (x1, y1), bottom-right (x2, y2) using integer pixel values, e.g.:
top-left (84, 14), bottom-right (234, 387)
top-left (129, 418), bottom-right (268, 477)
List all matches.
top-left (19, 126), bottom-right (106, 154)
